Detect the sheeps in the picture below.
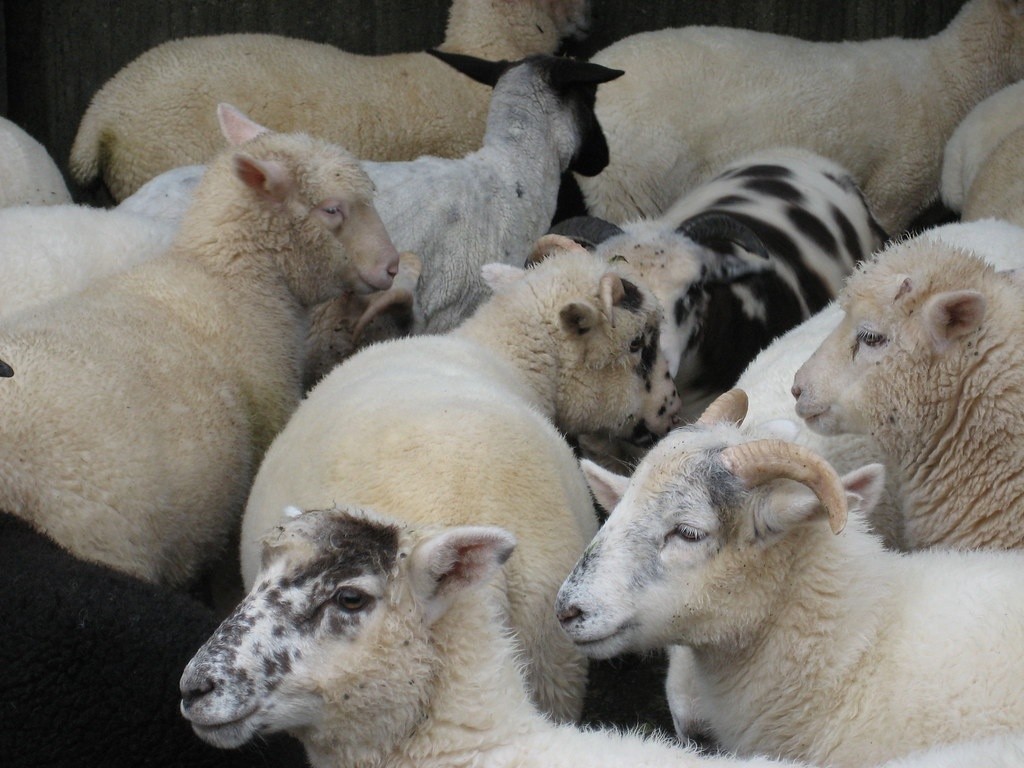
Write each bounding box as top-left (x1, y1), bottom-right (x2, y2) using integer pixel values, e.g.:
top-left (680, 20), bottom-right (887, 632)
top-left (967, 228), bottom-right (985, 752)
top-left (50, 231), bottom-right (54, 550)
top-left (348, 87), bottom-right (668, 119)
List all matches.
top-left (0, 357), bottom-right (309, 767)
top-left (177, 507), bottom-right (806, 767)
top-left (555, 389), bottom-right (1023, 767)
top-left (0, 1), bottom-right (1023, 724)
